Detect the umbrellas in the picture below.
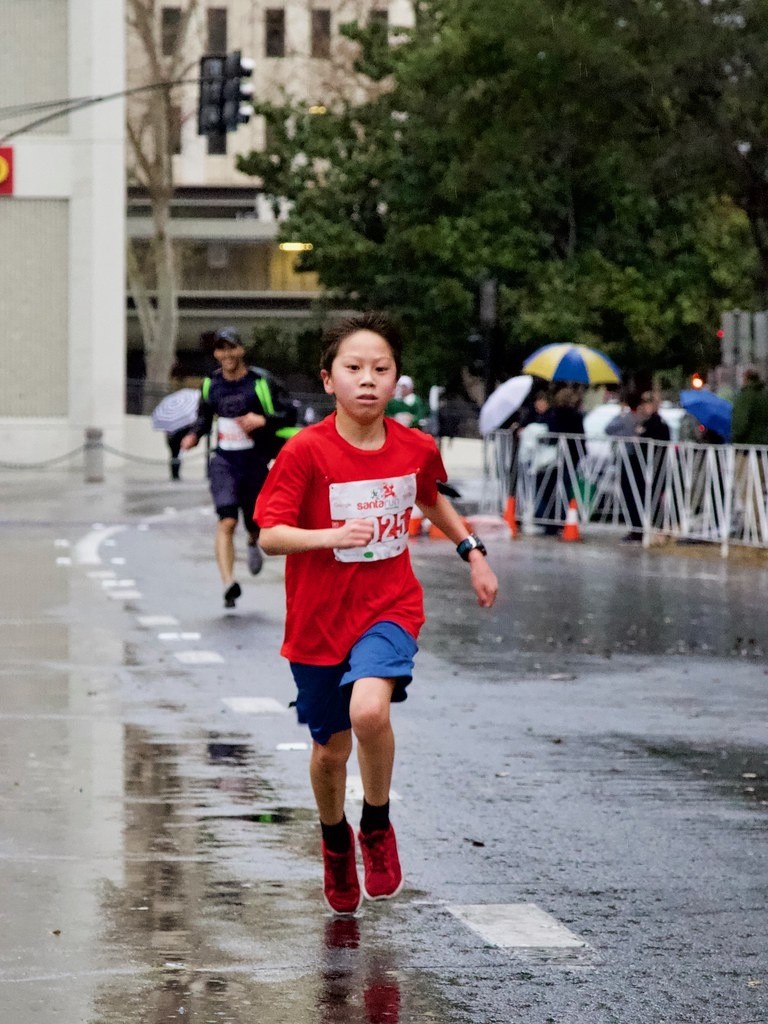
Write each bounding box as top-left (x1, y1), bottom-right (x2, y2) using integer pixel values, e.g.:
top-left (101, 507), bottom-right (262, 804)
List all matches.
top-left (478, 366), bottom-right (532, 438)
top-left (521, 341), bottom-right (626, 383)
top-left (680, 384), bottom-right (740, 437)
top-left (146, 386), bottom-right (204, 431)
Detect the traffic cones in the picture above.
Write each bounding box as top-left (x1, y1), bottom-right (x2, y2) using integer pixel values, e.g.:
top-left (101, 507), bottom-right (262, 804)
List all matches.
top-left (560, 498), bottom-right (581, 544)
top-left (504, 496), bottom-right (518, 540)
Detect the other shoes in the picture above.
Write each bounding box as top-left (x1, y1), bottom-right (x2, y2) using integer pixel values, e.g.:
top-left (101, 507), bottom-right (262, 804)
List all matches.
top-left (619, 529), bottom-right (643, 543)
top-left (223, 582), bottom-right (241, 609)
top-left (247, 546), bottom-right (264, 576)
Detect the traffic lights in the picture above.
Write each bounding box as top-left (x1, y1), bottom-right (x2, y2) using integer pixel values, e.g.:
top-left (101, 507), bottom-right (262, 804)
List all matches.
top-left (221, 50), bottom-right (253, 133)
top-left (685, 324), bottom-right (705, 391)
top-left (456, 280), bottom-right (476, 333)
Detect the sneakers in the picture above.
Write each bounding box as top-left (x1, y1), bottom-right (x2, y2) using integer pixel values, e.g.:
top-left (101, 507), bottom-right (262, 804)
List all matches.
top-left (359, 819), bottom-right (405, 902)
top-left (321, 822), bottom-right (363, 916)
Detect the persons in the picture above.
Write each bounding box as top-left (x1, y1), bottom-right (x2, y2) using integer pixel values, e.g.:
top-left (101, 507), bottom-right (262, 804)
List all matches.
top-left (385, 375), bottom-right (462, 430)
top-left (166, 425), bottom-right (196, 480)
top-left (251, 315), bottom-right (500, 916)
top-left (501, 368), bottom-right (768, 547)
top-left (177, 326), bottom-right (300, 610)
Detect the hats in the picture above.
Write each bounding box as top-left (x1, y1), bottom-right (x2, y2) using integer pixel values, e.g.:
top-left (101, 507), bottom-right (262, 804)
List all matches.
top-left (211, 325), bottom-right (241, 348)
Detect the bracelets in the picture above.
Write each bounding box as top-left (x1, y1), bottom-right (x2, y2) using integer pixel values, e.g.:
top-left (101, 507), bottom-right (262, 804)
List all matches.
top-left (455, 533), bottom-right (488, 564)
